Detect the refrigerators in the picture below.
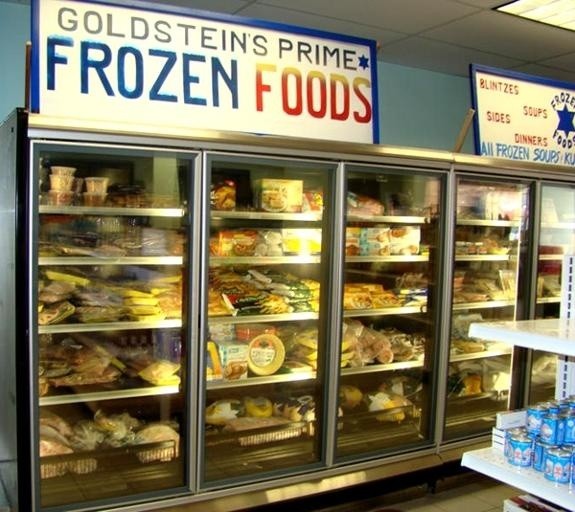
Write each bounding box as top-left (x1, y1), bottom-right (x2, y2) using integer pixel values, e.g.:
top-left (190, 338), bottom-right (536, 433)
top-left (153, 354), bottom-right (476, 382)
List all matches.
top-left (15, 110), bottom-right (575, 512)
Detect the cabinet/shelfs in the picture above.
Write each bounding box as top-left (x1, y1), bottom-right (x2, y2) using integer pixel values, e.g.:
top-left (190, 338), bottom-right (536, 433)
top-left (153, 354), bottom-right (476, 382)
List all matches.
top-left (523, 179), bottom-right (572, 418)
top-left (443, 169), bottom-right (534, 456)
top-left (28, 134), bottom-right (199, 510)
top-left (200, 147), bottom-right (337, 495)
top-left (330, 157), bottom-right (449, 471)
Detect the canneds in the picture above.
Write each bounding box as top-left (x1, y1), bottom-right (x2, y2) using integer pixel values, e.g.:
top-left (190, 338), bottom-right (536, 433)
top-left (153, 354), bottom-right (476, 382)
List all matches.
top-left (504, 394), bottom-right (575, 484)
